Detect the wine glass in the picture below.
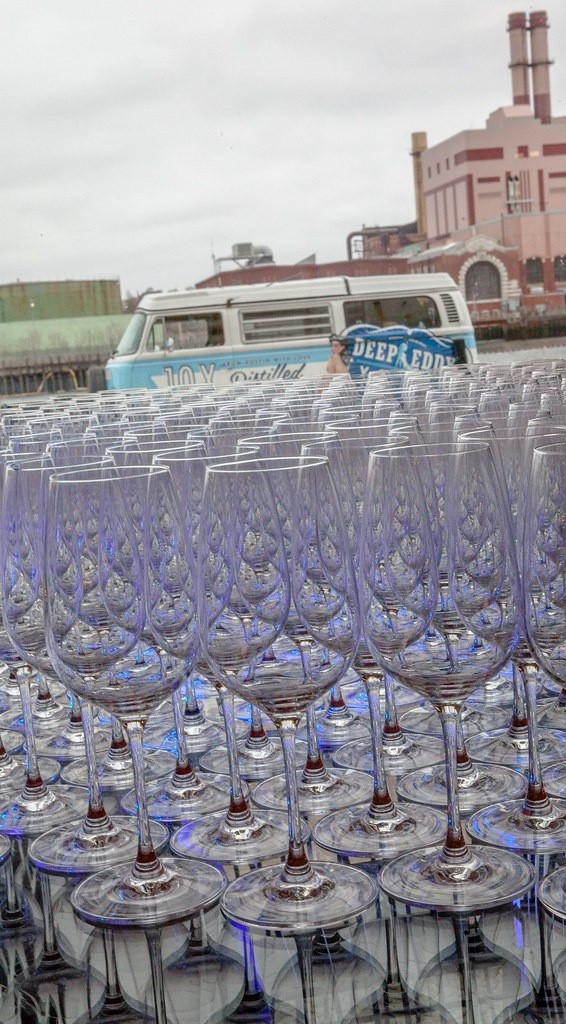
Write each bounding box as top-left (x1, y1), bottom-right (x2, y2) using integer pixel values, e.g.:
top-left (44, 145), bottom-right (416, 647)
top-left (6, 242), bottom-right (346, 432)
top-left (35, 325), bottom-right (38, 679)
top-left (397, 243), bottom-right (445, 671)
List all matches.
top-left (0, 834), bottom-right (565, 1024)
top-left (0, 357), bottom-right (566, 927)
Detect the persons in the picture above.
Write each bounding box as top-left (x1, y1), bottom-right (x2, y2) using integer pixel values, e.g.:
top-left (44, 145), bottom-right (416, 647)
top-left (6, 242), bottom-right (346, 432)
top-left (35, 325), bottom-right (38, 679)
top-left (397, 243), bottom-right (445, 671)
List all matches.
top-left (327, 334), bottom-right (350, 373)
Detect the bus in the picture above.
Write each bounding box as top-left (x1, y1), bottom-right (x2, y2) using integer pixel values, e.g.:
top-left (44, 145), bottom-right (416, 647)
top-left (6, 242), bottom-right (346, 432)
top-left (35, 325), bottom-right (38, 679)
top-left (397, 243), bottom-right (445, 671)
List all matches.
top-left (102, 272), bottom-right (478, 392)
